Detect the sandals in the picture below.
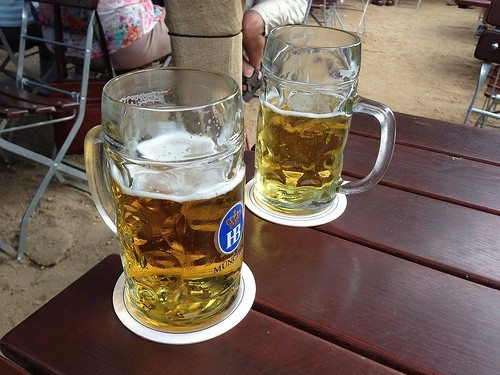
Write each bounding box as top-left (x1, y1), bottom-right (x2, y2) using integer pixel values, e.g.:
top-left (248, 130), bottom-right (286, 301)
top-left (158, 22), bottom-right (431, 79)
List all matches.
top-left (249, 59), bottom-right (265, 72)
top-left (370, 0), bottom-right (383, 6)
top-left (242, 70), bottom-right (262, 102)
top-left (386, 0), bottom-right (394, 6)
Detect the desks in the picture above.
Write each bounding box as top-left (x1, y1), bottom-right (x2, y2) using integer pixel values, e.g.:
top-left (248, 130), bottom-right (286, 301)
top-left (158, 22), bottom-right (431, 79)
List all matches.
top-left (0, 100), bottom-right (500, 375)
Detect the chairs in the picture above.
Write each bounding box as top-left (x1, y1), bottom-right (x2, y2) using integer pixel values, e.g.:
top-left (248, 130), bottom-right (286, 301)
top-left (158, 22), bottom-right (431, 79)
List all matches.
top-left (464, 0), bottom-right (500, 128)
top-left (0, 0), bottom-right (99, 263)
top-left (304, 0), bottom-right (369, 34)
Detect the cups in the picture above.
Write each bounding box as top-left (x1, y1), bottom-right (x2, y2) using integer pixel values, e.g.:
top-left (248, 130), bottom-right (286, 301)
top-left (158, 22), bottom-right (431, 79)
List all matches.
top-left (83, 67), bottom-right (258, 345)
top-left (243, 24), bottom-right (397, 228)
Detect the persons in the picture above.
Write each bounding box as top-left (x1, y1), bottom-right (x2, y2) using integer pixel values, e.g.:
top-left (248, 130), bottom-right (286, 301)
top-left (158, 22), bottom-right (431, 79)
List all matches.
top-left (242, 0), bottom-right (309, 102)
top-left (370, 0), bottom-right (396, 6)
top-left (0, 0), bottom-right (172, 72)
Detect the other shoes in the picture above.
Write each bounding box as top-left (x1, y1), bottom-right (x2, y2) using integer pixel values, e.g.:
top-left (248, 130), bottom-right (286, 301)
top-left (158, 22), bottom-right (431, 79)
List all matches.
top-left (39, 73), bottom-right (68, 83)
